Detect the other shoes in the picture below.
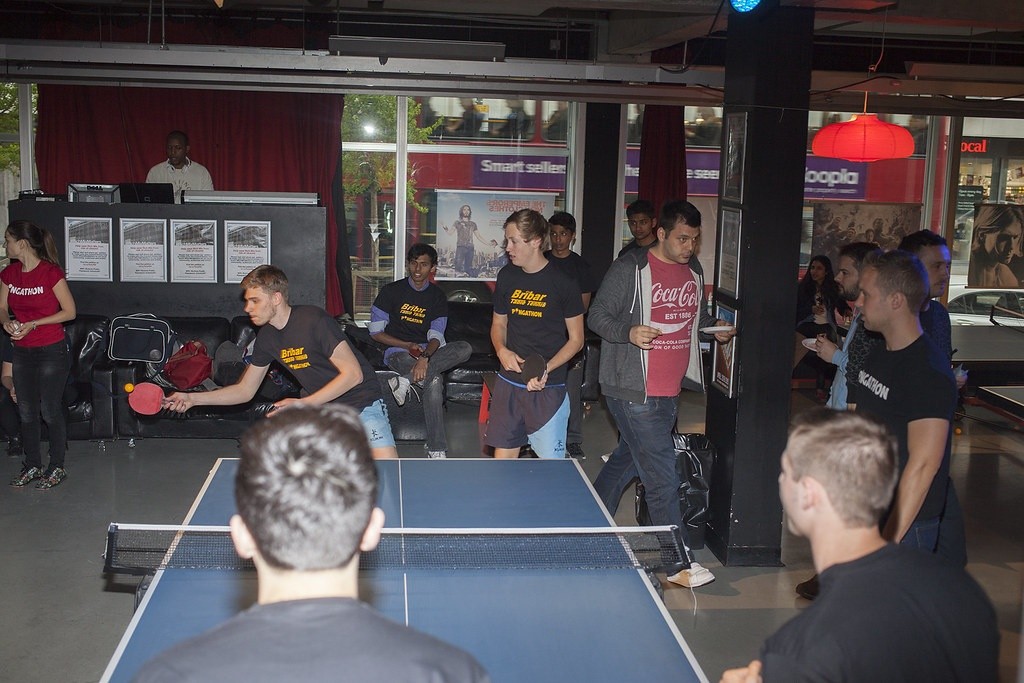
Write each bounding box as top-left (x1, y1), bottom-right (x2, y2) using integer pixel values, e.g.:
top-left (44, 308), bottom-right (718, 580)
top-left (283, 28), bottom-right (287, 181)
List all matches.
top-left (815, 390), bottom-right (827, 402)
top-left (795, 574), bottom-right (820, 601)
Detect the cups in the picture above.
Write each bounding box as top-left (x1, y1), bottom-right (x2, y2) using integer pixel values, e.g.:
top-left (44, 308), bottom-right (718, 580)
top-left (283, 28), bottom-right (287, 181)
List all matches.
top-left (844, 312), bottom-right (853, 325)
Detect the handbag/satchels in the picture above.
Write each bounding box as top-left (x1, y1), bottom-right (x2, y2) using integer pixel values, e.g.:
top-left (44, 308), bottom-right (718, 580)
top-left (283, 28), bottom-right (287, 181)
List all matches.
top-left (106, 308), bottom-right (244, 392)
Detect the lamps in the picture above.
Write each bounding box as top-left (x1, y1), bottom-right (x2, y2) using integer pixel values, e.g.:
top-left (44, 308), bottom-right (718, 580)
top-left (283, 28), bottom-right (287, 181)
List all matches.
top-left (811, 91), bottom-right (915, 163)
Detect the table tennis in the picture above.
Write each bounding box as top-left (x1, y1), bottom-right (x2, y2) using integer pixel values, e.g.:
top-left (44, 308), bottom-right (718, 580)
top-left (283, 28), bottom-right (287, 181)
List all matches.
top-left (125, 383), bottom-right (134, 392)
top-left (21, 323), bottom-right (24, 326)
top-left (14, 331), bottom-right (20, 335)
top-left (14, 324), bottom-right (19, 330)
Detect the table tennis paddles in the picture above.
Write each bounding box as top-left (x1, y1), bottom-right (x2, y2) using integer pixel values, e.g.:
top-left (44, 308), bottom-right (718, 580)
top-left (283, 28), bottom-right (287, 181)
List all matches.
top-left (128, 382), bottom-right (174, 416)
top-left (518, 353), bottom-right (547, 385)
top-left (409, 349), bottom-right (421, 358)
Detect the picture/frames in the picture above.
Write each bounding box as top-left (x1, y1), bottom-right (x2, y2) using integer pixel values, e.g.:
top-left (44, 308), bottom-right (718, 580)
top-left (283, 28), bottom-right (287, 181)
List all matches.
top-left (64, 217), bottom-right (112, 282)
top-left (119, 217), bottom-right (167, 282)
top-left (224, 220), bottom-right (271, 284)
top-left (720, 111), bottom-right (747, 205)
top-left (712, 303), bottom-right (737, 398)
top-left (170, 219), bottom-right (217, 283)
top-left (717, 205), bottom-right (742, 300)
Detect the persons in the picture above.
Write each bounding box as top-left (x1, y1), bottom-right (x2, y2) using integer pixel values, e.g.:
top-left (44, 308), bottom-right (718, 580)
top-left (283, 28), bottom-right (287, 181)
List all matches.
top-left (901, 115), bottom-right (929, 157)
top-left (968, 204), bottom-right (1023, 288)
top-left (546, 101), bottom-right (568, 141)
top-left (587, 200), bottom-right (737, 588)
top-left (500, 99), bottom-right (531, 139)
top-left (128, 406), bottom-right (491, 683)
top-left (720, 407), bottom-right (1001, 683)
top-left (685, 107), bottom-right (722, 146)
top-left (441, 204), bottom-right (494, 277)
top-left (0, 219), bottom-right (76, 491)
top-left (455, 98), bottom-right (483, 138)
top-left (816, 204), bottom-right (918, 268)
top-left (145, 130), bottom-right (215, 203)
top-left (164, 265), bottom-right (399, 459)
top-left (368, 198), bottom-right (659, 460)
top-left (797, 230), bottom-right (969, 608)
top-left (629, 104), bottom-right (644, 141)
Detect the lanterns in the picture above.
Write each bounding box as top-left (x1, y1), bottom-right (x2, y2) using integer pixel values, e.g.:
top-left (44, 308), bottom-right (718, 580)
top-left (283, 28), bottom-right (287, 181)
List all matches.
top-left (812, 114), bottom-right (915, 166)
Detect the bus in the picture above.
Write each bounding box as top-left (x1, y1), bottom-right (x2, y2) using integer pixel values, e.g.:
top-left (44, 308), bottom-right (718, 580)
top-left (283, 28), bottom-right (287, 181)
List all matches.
top-left (384, 100), bottom-right (951, 303)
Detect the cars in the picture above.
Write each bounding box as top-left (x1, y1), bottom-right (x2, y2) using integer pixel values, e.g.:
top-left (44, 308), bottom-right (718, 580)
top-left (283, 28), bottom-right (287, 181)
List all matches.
top-left (947, 288), bottom-right (1024, 326)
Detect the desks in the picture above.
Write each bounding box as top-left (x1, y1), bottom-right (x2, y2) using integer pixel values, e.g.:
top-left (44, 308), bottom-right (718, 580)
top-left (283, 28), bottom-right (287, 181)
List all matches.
top-left (979, 387), bottom-right (1024, 420)
top-left (100, 458), bottom-right (707, 683)
top-left (950, 328), bottom-right (1024, 365)
top-left (8, 199), bottom-right (327, 320)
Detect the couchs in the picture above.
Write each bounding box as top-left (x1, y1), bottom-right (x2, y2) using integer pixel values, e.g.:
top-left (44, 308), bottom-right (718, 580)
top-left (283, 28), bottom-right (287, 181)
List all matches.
top-left (444, 300), bottom-right (600, 405)
top-left (0, 316), bottom-right (114, 441)
top-left (115, 316), bottom-right (427, 446)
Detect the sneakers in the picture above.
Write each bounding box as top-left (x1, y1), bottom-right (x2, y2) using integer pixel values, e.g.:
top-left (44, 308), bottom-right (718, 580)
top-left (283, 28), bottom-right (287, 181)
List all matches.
top-left (517, 445), bottom-right (539, 458)
top-left (423, 442), bottom-right (448, 459)
top-left (7, 433), bottom-right (24, 457)
top-left (8, 460), bottom-right (44, 488)
top-left (565, 443), bottom-right (586, 460)
top-left (666, 561), bottom-right (715, 588)
top-left (388, 375), bottom-right (421, 406)
top-left (34, 464), bottom-right (68, 491)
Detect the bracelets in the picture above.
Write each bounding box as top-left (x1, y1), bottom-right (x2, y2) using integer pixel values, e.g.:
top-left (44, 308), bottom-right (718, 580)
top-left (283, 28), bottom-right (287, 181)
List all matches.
top-left (32, 320), bottom-right (36, 330)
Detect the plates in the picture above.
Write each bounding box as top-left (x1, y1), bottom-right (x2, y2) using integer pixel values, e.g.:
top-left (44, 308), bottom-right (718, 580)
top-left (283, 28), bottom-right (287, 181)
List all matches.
top-left (802, 337), bottom-right (839, 352)
top-left (699, 325), bottom-right (735, 334)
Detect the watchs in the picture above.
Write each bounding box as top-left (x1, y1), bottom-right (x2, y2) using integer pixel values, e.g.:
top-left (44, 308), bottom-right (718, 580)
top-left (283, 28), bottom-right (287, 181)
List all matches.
top-left (420, 351), bottom-right (431, 362)
